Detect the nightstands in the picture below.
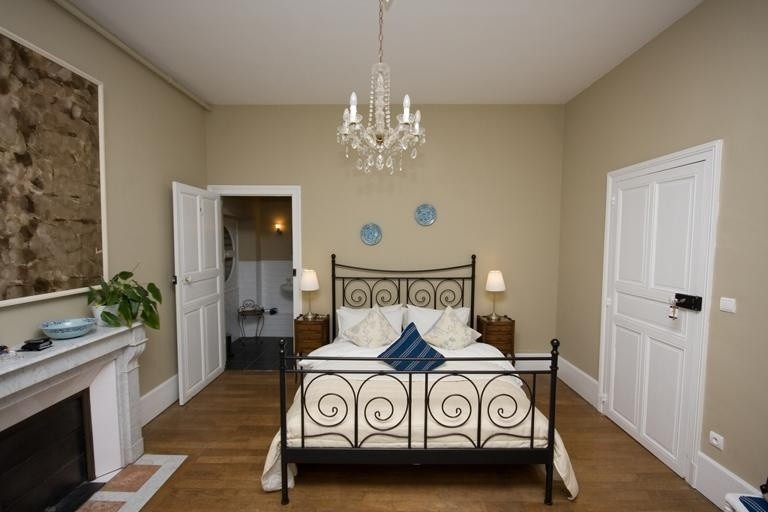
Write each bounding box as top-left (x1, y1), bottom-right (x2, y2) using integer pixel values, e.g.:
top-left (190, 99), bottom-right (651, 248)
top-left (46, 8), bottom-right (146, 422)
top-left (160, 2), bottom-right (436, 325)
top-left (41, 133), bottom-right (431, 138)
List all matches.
top-left (475, 314), bottom-right (515, 367)
top-left (295, 313), bottom-right (330, 356)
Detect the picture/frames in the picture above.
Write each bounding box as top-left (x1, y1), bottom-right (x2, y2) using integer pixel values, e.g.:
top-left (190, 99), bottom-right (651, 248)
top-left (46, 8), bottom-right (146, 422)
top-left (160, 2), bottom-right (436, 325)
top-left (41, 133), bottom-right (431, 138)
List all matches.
top-left (0, 26), bottom-right (110, 309)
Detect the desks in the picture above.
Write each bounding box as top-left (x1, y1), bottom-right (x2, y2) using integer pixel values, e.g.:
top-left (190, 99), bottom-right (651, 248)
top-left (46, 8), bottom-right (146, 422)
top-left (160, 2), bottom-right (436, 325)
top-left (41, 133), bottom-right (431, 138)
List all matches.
top-left (0, 318), bottom-right (149, 480)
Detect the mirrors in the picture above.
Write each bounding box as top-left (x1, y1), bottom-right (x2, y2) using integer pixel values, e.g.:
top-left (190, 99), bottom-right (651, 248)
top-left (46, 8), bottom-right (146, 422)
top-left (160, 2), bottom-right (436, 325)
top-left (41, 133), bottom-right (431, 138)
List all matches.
top-left (224, 226), bottom-right (234, 283)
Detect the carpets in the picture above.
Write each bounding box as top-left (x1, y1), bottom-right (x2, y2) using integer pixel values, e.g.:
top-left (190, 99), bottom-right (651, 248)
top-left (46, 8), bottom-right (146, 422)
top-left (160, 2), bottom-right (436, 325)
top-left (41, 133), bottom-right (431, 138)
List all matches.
top-left (74, 453), bottom-right (188, 512)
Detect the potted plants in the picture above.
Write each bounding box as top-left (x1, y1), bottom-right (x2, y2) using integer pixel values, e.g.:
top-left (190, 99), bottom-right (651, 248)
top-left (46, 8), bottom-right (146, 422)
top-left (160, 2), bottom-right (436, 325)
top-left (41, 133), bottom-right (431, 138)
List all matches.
top-left (90, 271), bottom-right (162, 327)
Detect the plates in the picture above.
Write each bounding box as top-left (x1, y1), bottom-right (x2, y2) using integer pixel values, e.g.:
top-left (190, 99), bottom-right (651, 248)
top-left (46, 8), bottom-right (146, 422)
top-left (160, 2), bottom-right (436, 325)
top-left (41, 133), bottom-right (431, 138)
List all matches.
top-left (359, 223), bottom-right (382, 245)
top-left (38, 318), bottom-right (99, 340)
top-left (415, 204), bottom-right (437, 226)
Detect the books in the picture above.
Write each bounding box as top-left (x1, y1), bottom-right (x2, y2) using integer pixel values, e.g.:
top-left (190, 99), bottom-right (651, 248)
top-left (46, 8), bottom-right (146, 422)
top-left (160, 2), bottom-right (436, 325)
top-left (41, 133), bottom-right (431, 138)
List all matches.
top-left (21, 337), bottom-right (53, 351)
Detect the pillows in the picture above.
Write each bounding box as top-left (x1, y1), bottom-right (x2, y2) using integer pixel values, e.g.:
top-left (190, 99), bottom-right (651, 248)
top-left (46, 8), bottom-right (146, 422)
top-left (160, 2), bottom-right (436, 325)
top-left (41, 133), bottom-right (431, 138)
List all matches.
top-left (421, 306), bottom-right (482, 351)
top-left (407, 303), bottom-right (470, 338)
top-left (378, 323), bottom-right (445, 373)
top-left (336, 304), bottom-right (402, 339)
top-left (343, 305), bottom-right (399, 350)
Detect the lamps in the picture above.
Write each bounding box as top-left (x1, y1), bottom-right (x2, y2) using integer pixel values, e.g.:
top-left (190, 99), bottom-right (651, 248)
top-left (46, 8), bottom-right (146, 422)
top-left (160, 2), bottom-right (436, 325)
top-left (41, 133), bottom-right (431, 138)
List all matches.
top-left (337, 1), bottom-right (427, 176)
top-left (485, 270), bottom-right (507, 319)
top-left (274, 223), bottom-right (283, 234)
top-left (301, 269), bottom-right (320, 318)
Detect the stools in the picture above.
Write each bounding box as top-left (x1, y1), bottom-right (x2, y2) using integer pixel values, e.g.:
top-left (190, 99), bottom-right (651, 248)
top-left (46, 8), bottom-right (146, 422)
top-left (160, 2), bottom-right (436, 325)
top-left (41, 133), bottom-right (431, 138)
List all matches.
top-left (238, 299), bottom-right (264, 340)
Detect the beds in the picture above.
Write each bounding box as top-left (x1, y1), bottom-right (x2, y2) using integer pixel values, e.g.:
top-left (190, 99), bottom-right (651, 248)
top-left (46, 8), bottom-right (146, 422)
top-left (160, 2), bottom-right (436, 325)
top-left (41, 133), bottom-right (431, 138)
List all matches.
top-left (279, 254), bottom-right (560, 505)
